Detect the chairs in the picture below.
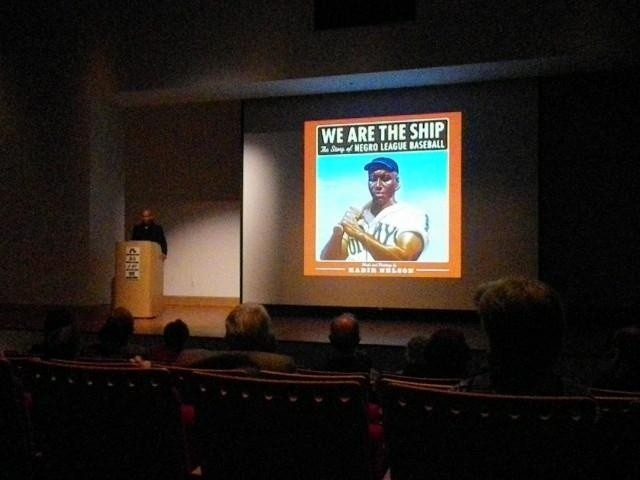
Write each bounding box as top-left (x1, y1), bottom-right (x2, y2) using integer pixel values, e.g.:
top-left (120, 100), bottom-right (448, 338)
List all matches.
top-left (378, 375), bottom-right (639, 479)
top-left (3, 356), bottom-right (200, 480)
top-left (4, 349), bottom-right (638, 400)
top-left (172, 366), bottom-right (387, 479)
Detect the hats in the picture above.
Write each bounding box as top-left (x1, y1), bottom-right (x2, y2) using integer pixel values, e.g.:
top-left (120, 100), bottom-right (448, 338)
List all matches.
top-left (362, 156), bottom-right (399, 174)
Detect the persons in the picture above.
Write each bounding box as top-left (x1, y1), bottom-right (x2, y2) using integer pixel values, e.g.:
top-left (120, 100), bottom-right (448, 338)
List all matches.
top-left (309, 311), bottom-right (375, 374)
top-left (597, 325), bottom-right (640, 393)
top-left (222, 301), bottom-right (272, 350)
top-left (30, 320), bottom-right (81, 359)
top-left (398, 334), bottom-right (433, 377)
top-left (548, 365), bottom-right (591, 398)
top-left (473, 276), bottom-right (566, 400)
top-left (148, 318), bottom-right (190, 363)
top-left (315, 157), bottom-right (431, 264)
top-left (424, 328), bottom-right (472, 383)
top-left (93, 306), bottom-right (140, 362)
top-left (130, 208), bottom-right (167, 255)
